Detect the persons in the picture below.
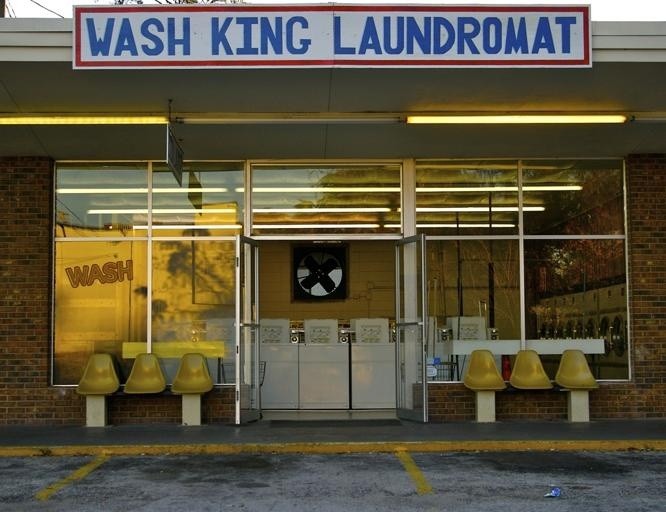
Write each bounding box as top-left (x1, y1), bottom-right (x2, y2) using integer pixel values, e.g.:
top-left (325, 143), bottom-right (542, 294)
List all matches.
top-left (132, 285), bottom-right (152, 306)
top-left (148, 299), bottom-right (177, 341)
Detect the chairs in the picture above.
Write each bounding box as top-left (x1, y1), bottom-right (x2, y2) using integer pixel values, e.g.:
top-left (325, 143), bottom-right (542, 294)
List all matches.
top-left (75, 352), bottom-right (267, 427)
top-left (401, 349), bottom-right (600, 423)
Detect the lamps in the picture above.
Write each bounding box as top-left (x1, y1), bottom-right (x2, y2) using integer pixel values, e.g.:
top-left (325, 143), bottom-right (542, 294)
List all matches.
top-left (174, 115), bottom-right (403, 126)
top-left (1, 115), bottom-right (171, 125)
top-left (630, 115), bottom-right (666, 126)
top-left (405, 114), bottom-right (630, 125)
